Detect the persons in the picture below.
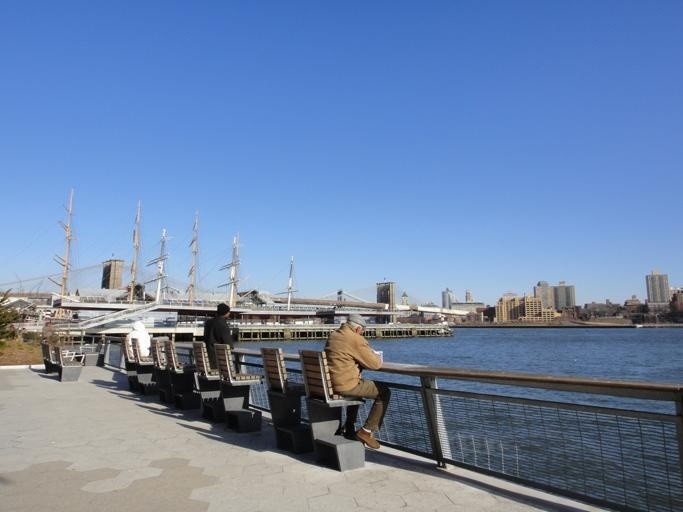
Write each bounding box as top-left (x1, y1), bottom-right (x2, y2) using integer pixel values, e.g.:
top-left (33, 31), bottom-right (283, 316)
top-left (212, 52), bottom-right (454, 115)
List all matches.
top-left (126, 322), bottom-right (151, 357)
top-left (324, 314), bottom-right (391, 449)
top-left (203, 303), bottom-right (241, 373)
top-left (42, 321), bottom-right (52, 340)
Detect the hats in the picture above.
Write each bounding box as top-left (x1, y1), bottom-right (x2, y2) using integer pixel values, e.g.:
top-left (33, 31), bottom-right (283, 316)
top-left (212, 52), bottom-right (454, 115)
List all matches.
top-left (349, 314), bottom-right (366, 328)
top-left (218, 304), bottom-right (230, 316)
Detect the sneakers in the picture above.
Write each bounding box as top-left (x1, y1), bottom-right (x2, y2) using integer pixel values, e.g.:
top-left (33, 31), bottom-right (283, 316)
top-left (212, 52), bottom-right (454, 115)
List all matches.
top-left (356, 427), bottom-right (381, 449)
top-left (341, 424), bottom-right (355, 436)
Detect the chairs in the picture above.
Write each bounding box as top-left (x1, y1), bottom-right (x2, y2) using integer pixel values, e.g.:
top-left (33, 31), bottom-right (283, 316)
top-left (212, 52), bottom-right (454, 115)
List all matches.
top-left (121, 338), bottom-right (367, 472)
top-left (41, 341), bottom-right (85, 381)
